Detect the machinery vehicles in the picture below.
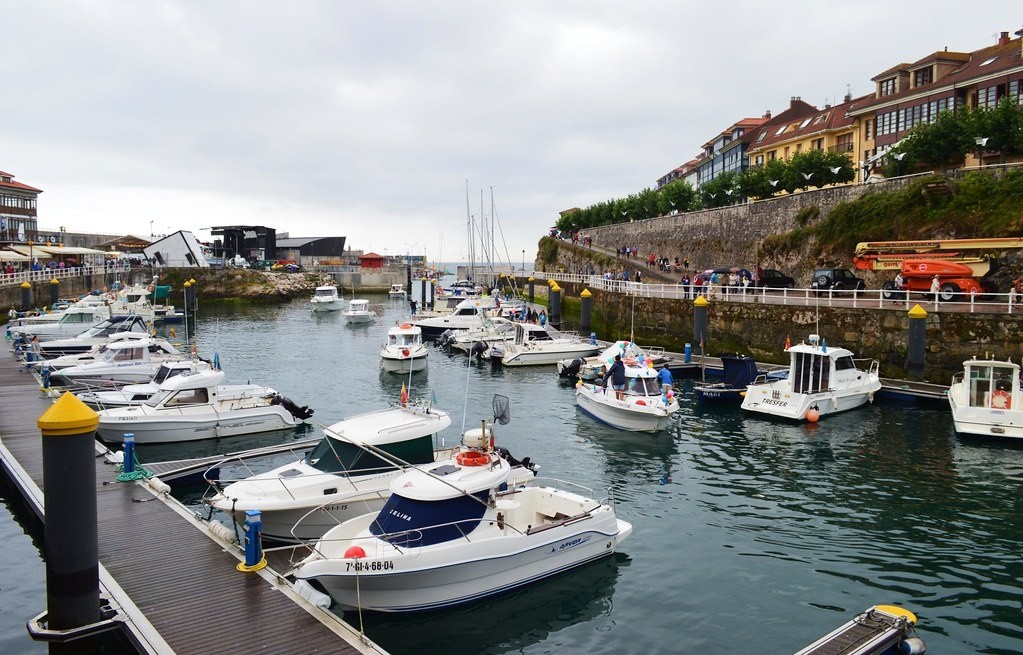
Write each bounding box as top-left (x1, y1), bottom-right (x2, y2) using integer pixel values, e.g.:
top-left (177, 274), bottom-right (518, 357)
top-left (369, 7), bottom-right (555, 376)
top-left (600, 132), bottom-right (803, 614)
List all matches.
top-left (854, 238), bottom-right (1023, 302)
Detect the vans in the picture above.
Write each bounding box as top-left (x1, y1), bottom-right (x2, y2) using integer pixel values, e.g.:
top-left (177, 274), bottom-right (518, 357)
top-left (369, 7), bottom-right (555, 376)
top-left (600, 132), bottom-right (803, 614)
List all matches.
top-left (253, 259), bottom-right (278, 270)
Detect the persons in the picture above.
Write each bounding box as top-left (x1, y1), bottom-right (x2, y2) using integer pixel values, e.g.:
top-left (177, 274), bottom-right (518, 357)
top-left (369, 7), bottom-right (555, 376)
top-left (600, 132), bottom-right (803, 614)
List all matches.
top-left (893, 271), bottom-right (906, 305)
top-left (410, 299), bottom-right (417, 315)
top-left (599, 355), bottom-right (626, 401)
top-left (549, 229), bottom-right (592, 249)
top-left (653, 364), bottom-right (674, 406)
top-left (682, 270), bottom-right (747, 299)
top-left (930, 274), bottom-right (944, 305)
top-left (4, 257), bottom-right (141, 283)
top-left (616, 243), bottom-right (689, 273)
top-left (31, 335), bottom-right (42, 372)
top-left (526, 307), bottom-right (547, 328)
top-left (556, 266), bottom-right (641, 292)
top-left (8, 306), bottom-right (18, 319)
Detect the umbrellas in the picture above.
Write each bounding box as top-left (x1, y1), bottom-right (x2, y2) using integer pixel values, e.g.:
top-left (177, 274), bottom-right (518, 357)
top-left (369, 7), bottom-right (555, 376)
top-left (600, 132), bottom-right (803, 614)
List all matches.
top-left (696, 267), bottom-right (753, 281)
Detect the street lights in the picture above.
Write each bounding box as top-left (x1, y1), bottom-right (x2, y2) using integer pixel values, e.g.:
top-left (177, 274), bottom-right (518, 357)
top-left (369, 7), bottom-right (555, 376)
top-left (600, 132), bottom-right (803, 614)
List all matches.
top-left (27, 240), bottom-right (33, 270)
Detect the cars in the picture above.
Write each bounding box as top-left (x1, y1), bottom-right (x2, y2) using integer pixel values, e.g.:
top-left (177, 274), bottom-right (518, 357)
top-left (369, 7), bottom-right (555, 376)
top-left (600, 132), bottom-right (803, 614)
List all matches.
top-left (746, 269), bottom-right (795, 295)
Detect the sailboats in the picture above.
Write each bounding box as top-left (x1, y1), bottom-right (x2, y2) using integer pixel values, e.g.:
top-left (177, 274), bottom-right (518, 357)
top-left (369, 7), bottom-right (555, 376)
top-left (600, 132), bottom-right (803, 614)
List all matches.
top-left (439, 174), bottom-right (529, 317)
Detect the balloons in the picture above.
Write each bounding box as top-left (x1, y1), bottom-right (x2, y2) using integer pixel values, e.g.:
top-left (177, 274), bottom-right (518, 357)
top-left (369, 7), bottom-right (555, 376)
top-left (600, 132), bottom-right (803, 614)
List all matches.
top-left (620, 341), bottom-right (628, 348)
top-left (638, 356), bottom-right (653, 369)
top-left (608, 358), bottom-right (614, 364)
top-left (662, 390), bottom-right (674, 403)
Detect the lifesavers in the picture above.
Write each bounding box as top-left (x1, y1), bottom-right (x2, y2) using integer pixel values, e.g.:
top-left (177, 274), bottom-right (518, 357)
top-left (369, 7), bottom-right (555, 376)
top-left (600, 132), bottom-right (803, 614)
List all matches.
top-left (400, 324), bottom-right (411, 329)
top-left (620, 357), bottom-right (639, 365)
top-left (105, 293), bottom-right (115, 300)
top-left (988, 390), bottom-right (1011, 409)
top-left (436, 287), bottom-right (444, 296)
top-left (147, 285), bottom-right (155, 292)
top-left (455, 451), bottom-right (491, 466)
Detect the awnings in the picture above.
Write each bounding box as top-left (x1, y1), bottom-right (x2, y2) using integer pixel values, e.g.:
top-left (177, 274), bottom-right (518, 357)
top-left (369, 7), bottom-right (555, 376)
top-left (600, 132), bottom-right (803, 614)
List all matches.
top-left (0, 246), bottom-right (122, 262)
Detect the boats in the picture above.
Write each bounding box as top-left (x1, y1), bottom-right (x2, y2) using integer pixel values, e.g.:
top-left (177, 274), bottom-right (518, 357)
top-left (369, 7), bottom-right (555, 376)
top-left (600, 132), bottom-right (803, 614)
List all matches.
top-left (406, 298), bottom-right (607, 368)
top-left (7, 280), bottom-right (316, 444)
top-left (691, 355), bottom-right (790, 405)
top-left (948, 353), bottom-right (1023, 443)
top-left (573, 295), bottom-right (681, 433)
top-left (200, 378), bottom-right (461, 550)
top-left (378, 323), bottom-right (430, 375)
top-left (342, 298), bottom-right (377, 324)
top-left (287, 341), bottom-right (629, 615)
top-left (389, 284), bottom-right (407, 299)
top-left (739, 332), bottom-right (882, 423)
top-left (310, 286), bottom-right (348, 312)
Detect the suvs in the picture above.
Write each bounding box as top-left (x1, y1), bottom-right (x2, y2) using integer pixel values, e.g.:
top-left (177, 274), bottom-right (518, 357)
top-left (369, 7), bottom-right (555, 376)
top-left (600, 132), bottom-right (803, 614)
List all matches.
top-left (808, 269), bottom-right (867, 298)
